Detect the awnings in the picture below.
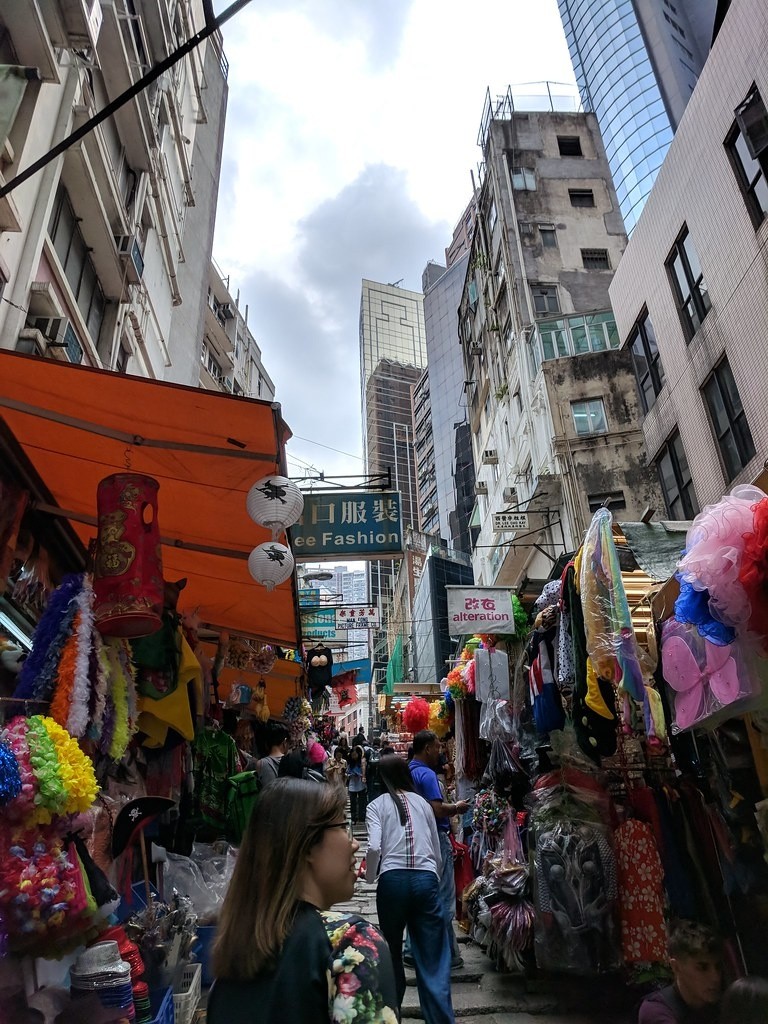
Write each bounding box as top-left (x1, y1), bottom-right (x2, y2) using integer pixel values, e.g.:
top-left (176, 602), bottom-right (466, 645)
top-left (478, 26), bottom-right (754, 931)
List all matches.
top-left (208, 650), bottom-right (304, 725)
top-left (2, 345), bottom-right (304, 651)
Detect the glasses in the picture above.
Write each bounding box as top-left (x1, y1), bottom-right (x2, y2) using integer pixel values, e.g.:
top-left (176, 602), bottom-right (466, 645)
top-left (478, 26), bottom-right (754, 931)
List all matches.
top-left (326, 821), bottom-right (354, 840)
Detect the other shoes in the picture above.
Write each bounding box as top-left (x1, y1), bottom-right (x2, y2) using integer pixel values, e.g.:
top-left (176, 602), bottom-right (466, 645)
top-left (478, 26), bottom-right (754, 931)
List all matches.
top-left (403, 954), bottom-right (415, 966)
top-left (451, 957), bottom-right (465, 969)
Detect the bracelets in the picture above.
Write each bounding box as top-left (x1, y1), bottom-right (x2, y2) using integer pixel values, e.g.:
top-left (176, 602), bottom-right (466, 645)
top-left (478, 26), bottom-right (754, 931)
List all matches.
top-left (454, 805), bottom-right (460, 815)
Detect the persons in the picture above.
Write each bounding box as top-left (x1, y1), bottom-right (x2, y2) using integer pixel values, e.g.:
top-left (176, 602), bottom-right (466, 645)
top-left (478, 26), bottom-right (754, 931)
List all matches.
top-left (206, 779), bottom-right (364, 1024)
top-left (404, 730), bottom-right (475, 972)
top-left (635, 923), bottom-right (729, 1024)
top-left (256, 722), bottom-right (294, 792)
top-left (361, 753), bottom-right (458, 1024)
top-left (315, 720), bottom-right (451, 830)
top-left (717, 974), bottom-right (768, 1024)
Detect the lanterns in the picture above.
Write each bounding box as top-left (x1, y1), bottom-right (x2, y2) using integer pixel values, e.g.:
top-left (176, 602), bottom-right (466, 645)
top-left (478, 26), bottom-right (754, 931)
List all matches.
top-left (246, 473), bottom-right (304, 542)
top-left (94, 471), bottom-right (166, 639)
top-left (246, 542), bottom-right (296, 591)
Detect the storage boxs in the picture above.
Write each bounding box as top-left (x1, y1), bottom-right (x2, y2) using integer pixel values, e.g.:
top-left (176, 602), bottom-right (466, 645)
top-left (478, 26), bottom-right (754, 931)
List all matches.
top-left (115, 879), bottom-right (160, 924)
top-left (172, 963), bottom-right (203, 1024)
top-left (142, 984), bottom-right (175, 1024)
top-left (171, 922), bottom-right (220, 987)
